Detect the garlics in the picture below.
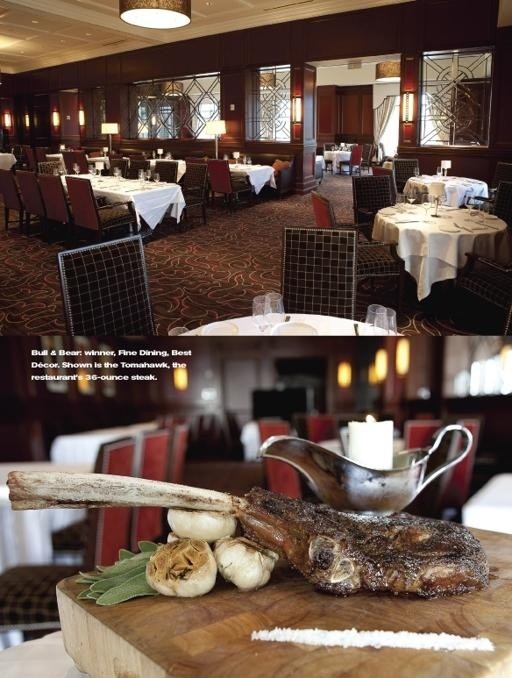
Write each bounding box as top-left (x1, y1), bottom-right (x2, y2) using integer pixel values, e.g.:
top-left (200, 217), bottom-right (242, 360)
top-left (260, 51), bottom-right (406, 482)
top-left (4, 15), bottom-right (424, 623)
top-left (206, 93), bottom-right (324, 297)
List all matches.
top-left (145, 505), bottom-right (279, 598)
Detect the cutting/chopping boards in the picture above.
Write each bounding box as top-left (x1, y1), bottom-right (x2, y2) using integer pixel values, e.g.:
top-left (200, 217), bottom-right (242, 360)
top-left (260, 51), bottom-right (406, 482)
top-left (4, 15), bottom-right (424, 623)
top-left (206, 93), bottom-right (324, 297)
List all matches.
top-left (54, 520), bottom-right (511, 678)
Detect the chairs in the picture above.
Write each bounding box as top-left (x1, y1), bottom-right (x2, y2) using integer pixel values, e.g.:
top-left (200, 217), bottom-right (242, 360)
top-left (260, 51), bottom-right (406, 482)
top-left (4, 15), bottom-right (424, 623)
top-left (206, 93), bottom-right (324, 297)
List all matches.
top-left (0, 138), bottom-right (512, 335)
top-left (0, 406), bottom-right (512, 677)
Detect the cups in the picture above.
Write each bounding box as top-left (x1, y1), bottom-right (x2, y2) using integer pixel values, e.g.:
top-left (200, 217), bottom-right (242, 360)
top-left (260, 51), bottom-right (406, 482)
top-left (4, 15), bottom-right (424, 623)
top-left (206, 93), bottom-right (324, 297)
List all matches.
top-left (365, 303), bottom-right (403, 336)
top-left (436, 167), bottom-right (442, 176)
top-left (414, 167), bottom-right (419, 178)
top-left (167, 327), bottom-right (191, 335)
top-left (252, 291), bottom-right (285, 336)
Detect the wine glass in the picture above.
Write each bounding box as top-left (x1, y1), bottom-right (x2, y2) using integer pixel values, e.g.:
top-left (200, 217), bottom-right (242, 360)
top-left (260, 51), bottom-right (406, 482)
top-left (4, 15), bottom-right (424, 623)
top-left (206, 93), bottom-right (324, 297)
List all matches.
top-left (52, 162), bottom-right (160, 186)
top-left (463, 191), bottom-right (475, 216)
top-left (406, 189), bottom-right (416, 206)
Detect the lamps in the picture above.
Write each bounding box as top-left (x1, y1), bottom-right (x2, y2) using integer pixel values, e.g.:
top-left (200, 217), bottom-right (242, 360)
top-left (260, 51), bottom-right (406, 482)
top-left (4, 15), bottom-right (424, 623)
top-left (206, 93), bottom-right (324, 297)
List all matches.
top-left (23, 111), bottom-right (30, 128)
top-left (120, 0), bottom-right (194, 30)
top-left (78, 107), bottom-right (84, 126)
top-left (205, 120), bottom-right (226, 159)
top-left (100, 122), bottom-right (120, 152)
top-left (2, 111), bottom-right (12, 128)
top-left (401, 88), bottom-right (416, 126)
top-left (289, 94), bottom-right (303, 126)
top-left (51, 110), bottom-right (60, 127)
top-left (374, 61), bottom-right (401, 85)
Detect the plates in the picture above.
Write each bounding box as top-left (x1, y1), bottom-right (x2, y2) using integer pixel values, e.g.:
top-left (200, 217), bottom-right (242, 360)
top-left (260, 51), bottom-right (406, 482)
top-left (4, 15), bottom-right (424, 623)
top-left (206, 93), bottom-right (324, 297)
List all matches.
top-left (377, 203), bottom-right (498, 232)
